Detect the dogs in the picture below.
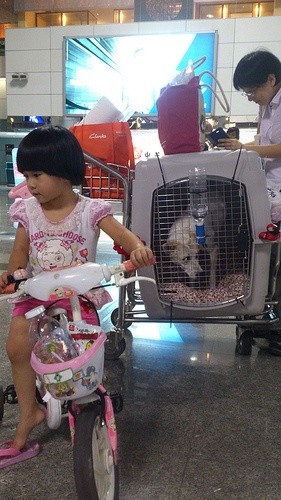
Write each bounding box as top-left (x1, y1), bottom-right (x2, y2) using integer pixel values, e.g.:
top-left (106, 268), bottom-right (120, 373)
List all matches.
top-left (162, 189), bottom-right (228, 290)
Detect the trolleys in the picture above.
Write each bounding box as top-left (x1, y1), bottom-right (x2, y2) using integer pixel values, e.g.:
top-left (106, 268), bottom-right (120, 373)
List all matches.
top-left (116, 154), bottom-right (281, 360)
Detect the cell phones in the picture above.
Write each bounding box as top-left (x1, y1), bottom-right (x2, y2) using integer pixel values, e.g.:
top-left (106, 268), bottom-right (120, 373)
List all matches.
top-left (205, 128), bottom-right (230, 146)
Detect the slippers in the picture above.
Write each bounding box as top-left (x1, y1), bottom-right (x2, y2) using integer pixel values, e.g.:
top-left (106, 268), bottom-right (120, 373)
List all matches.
top-left (0, 441), bottom-right (40, 468)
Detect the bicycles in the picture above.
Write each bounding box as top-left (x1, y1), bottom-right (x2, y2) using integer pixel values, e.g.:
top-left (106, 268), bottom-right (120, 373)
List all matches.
top-left (0, 257), bottom-right (158, 498)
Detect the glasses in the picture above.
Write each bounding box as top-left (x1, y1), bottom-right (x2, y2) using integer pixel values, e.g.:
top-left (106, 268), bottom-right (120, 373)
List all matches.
top-left (242, 85), bottom-right (259, 98)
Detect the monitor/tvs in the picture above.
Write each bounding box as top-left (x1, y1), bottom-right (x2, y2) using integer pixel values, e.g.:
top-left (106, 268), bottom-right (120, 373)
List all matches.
top-left (62, 31), bottom-right (218, 117)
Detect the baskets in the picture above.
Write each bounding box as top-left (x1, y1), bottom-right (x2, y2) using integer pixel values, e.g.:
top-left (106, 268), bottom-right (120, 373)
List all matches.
top-left (30, 332), bottom-right (107, 401)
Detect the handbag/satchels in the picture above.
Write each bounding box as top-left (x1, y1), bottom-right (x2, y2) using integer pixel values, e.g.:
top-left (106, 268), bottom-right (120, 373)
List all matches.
top-left (157, 57), bottom-right (229, 155)
top-left (69, 115), bottom-right (134, 200)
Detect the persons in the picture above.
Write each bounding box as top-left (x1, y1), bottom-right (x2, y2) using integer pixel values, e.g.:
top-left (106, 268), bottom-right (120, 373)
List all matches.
top-left (226, 127), bottom-right (239, 140)
top-left (9, 125), bottom-right (153, 454)
top-left (217, 48), bottom-right (281, 350)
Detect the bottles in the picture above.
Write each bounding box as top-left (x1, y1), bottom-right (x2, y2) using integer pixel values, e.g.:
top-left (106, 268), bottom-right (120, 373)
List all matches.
top-left (24, 305), bottom-right (79, 363)
top-left (188, 167), bottom-right (209, 244)
